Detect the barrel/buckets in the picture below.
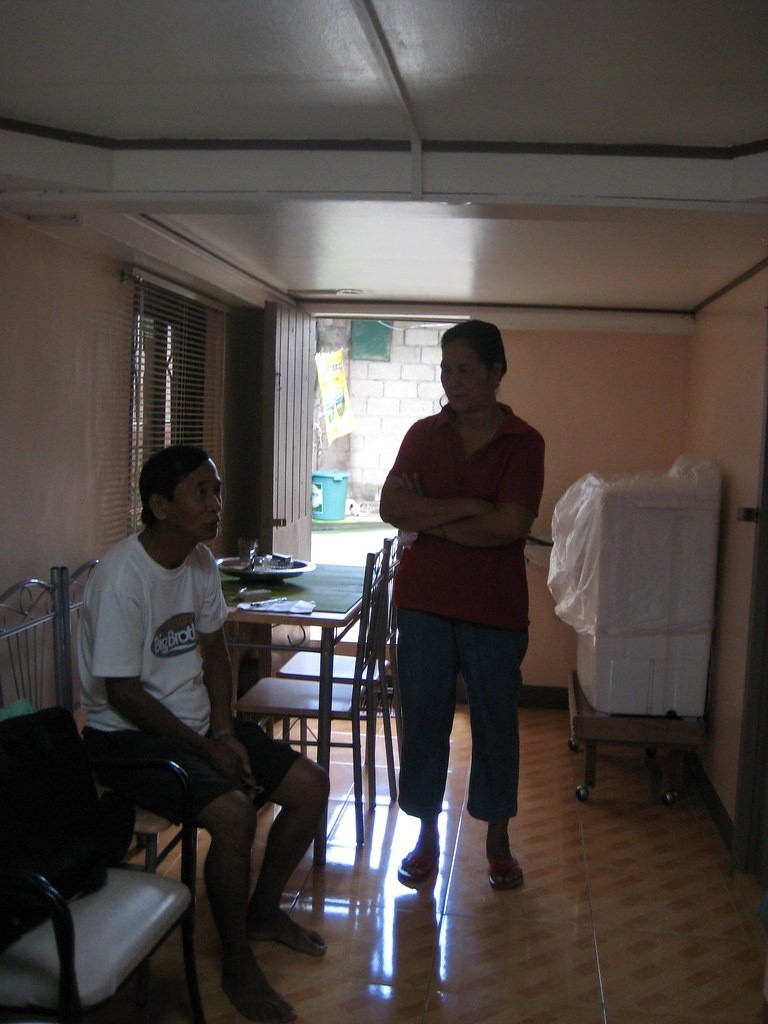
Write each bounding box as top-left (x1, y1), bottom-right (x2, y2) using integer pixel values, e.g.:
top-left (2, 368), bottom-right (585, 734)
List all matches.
top-left (312, 471), bottom-right (350, 520)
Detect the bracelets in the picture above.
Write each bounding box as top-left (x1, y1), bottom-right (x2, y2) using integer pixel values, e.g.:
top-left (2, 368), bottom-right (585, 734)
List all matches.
top-left (211, 728), bottom-right (233, 740)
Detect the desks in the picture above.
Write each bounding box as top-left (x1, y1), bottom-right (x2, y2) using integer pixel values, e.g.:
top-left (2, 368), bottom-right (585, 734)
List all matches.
top-left (219, 562), bottom-right (402, 867)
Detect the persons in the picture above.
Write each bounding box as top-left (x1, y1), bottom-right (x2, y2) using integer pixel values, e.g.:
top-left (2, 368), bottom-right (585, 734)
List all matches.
top-left (76, 445), bottom-right (332, 1024)
top-left (379, 320), bottom-right (545, 890)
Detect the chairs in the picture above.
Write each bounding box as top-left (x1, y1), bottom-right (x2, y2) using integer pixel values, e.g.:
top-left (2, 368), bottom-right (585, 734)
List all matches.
top-left (0, 536), bottom-right (402, 1024)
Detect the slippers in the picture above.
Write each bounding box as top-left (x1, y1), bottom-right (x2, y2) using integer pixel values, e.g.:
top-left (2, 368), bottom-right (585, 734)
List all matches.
top-left (399, 848), bottom-right (439, 882)
top-left (485, 848), bottom-right (523, 890)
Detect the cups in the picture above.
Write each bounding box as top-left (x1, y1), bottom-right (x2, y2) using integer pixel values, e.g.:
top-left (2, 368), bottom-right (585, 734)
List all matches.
top-left (237, 536), bottom-right (257, 568)
